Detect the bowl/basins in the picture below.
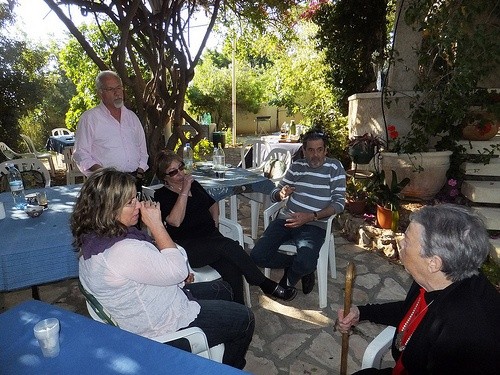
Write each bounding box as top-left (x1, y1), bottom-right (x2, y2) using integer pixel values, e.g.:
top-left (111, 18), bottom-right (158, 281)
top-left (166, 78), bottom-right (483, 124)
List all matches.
top-left (25, 193), bottom-right (37, 204)
top-left (195, 164), bottom-right (212, 175)
top-left (25, 206), bottom-right (44, 218)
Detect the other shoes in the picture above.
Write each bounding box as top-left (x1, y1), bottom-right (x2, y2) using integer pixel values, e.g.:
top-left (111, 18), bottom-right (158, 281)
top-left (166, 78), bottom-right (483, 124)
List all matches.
top-left (301, 273), bottom-right (315, 294)
top-left (279, 274), bottom-right (300, 289)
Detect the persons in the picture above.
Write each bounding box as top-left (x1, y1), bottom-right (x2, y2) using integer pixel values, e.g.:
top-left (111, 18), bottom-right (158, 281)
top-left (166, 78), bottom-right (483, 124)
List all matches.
top-left (337, 203), bottom-right (500, 375)
top-left (249, 129), bottom-right (347, 294)
top-left (69, 167), bottom-right (256, 370)
top-left (71, 71), bottom-right (151, 228)
top-left (153, 151), bottom-right (298, 304)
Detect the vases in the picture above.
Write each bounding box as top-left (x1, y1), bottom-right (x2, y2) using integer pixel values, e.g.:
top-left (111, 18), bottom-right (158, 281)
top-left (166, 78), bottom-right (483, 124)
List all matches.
top-left (346, 168), bottom-right (373, 188)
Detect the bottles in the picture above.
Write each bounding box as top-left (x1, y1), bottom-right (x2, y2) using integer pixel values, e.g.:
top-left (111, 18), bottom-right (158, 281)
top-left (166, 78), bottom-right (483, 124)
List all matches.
top-left (7, 163), bottom-right (27, 210)
top-left (213, 142), bottom-right (225, 172)
top-left (183, 143), bottom-right (195, 174)
top-left (290, 120), bottom-right (296, 135)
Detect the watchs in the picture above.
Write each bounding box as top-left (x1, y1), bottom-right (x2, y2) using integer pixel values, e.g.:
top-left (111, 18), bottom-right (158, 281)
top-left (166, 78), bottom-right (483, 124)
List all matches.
top-left (314, 211), bottom-right (318, 222)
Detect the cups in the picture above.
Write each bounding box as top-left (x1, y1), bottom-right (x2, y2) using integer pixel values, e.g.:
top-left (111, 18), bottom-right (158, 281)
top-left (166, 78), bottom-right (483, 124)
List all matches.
top-left (216, 172), bottom-right (225, 180)
top-left (35, 190), bottom-right (49, 211)
top-left (33, 318), bottom-right (60, 358)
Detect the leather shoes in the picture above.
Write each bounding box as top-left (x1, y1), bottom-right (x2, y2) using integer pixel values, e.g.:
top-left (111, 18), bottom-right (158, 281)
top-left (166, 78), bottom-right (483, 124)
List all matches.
top-left (265, 283), bottom-right (298, 302)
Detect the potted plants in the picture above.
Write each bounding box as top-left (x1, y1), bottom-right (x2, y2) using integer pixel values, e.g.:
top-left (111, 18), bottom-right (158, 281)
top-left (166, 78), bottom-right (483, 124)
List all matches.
top-left (376, 171), bottom-right (413, 229)
top-left (381, 41), bottom-right (450, 201)
top-left (347, 132), bottom-right (381, 163)
top-left (346, 183), bottom-right (367, 213)
top-left (461, 85), bottom-right (500, 139)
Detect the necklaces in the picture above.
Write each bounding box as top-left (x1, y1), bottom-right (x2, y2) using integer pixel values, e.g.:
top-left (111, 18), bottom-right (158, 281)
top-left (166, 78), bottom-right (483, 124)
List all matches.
top-left (394, 293), bottom-right (435, 351)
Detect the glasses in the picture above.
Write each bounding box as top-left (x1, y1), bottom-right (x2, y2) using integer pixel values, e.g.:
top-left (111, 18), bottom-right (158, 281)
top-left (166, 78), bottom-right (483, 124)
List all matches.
top-left (165, 161), bottom-right (185, 177)
top-left (121, 192), bottom-right (141, 209)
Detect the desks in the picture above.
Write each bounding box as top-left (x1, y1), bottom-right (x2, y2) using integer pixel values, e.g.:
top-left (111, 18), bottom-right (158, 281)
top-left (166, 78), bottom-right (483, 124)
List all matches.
top-left (192, 160), bottom-right (269, 220)
top-left (0, 299), bottom-right (252, 375)
top-left (0, 184), bottom-right (81, 300)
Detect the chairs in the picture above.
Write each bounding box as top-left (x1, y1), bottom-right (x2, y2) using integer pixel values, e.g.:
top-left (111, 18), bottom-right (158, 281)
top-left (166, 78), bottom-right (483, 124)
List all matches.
top-left (0, 128), bottom-right (82, 188)
top-left (78, 140), bottom-right (337, 362)
